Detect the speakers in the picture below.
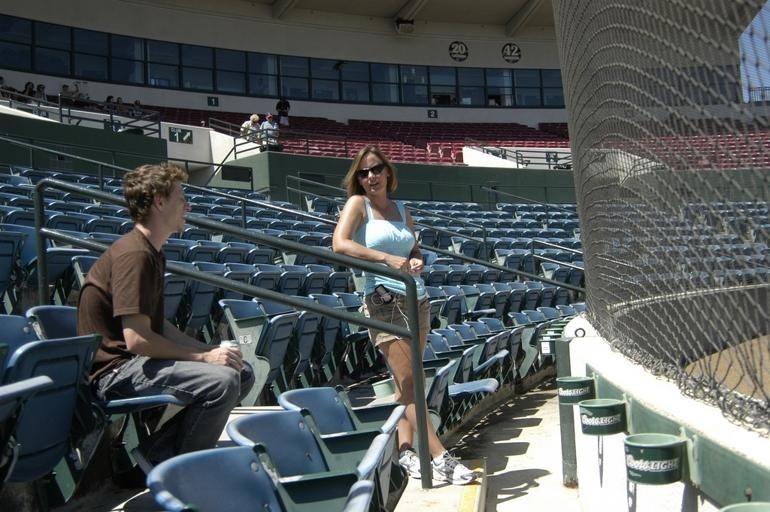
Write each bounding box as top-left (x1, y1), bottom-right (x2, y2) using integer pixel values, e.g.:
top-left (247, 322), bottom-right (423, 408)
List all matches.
top-left (399, 23), bottom-right (416, 35)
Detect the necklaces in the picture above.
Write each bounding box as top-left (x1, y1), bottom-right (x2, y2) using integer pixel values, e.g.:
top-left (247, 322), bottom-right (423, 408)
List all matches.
top-left (374, 201), bottom-right (392, 221)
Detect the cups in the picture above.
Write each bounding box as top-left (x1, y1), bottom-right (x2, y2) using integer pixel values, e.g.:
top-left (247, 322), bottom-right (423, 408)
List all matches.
top-left (219, 339), bottom-right (242, 397)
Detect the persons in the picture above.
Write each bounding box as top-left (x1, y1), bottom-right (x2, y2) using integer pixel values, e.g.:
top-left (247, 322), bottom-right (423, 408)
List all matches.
top-left (238, 113), bottom-right (261, 145)
top-left (0, 76), bottom-right (147, 121)
top-left (330, 143), bottom-right (480, 487)
top-left (72, 159), bottom-right (256, 481)
top-left (275, 97), bottom-right (291, 126)
top-left (256, 112), bottom-right (281, 147)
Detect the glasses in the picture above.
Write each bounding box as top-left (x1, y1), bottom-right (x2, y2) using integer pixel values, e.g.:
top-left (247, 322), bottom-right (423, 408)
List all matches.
top-left (357, 163), bottom-right (384, 179)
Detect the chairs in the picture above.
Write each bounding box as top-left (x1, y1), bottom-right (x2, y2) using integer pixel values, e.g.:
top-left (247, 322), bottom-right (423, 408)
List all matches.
top-left (0, 85), bottom-right (770, 512)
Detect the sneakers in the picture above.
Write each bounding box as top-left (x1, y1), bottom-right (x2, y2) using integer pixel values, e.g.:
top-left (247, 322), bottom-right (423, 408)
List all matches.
top-left (399, 450), bottom-right (477, 485)
top-left (131, 433), bottom-right (172, 476)
